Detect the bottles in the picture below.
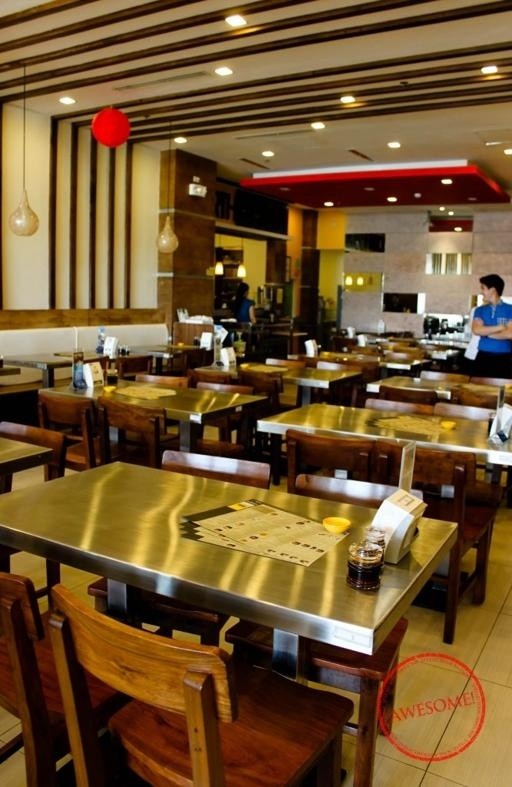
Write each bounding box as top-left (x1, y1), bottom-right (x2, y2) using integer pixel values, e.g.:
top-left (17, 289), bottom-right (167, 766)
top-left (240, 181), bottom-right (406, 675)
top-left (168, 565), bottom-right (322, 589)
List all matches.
top-left (345, 542), bottom-right (383, 592)
top-left (233, 332), bottom-right (246, 358)
top-left (106, 369), bottom-right (118, 386)
top-left (194, 336), bottom-right (200, 346)
top-left (0, 355), bottom-right (3, 368)
top-left (360, 527), bottom-right (385, 571)
top-left (488, 412), bottom-right (496, 438)
top-left (120, 345), bottom-right (130, 356)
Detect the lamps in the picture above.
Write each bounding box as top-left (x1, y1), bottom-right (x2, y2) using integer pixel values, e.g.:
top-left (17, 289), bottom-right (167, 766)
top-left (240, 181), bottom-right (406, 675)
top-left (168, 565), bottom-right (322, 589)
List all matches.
top-left (237, 238), bottom-right (246, 277)
top-left (156, 122), bottom-right (179, 252)
top-left (9, 64), bottom-right (40, 237)
top-left (214, 235), bottom-right (224, 275)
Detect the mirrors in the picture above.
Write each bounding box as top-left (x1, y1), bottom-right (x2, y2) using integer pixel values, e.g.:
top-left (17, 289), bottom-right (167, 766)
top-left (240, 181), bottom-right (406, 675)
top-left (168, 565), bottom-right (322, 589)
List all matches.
top-left (427, 208), bottom-right (474, 275)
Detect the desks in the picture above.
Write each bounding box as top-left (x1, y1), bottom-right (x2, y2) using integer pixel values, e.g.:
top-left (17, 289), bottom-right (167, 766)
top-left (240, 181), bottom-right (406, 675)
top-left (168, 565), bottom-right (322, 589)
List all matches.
top-left (0, 331), bottom-right (512, 787)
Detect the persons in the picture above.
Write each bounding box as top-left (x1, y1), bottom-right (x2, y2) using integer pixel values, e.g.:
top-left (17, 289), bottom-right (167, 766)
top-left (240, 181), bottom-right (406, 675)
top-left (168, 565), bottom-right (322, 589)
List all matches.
top-left (471, 273), bottom-right (512, 377)
top-left (229, 283), bottom-right (256, 324)
